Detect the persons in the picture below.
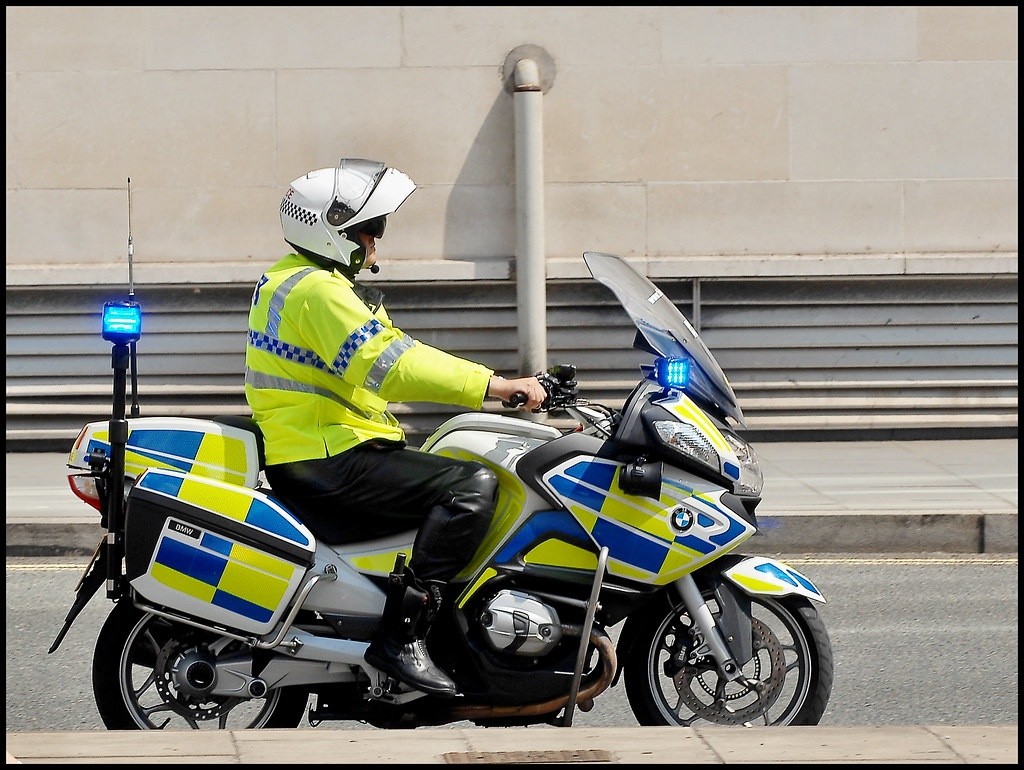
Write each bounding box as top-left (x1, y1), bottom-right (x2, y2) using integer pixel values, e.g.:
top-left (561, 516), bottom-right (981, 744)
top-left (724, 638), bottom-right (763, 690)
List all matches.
top-left (238, 158), bottom-right (551, 700)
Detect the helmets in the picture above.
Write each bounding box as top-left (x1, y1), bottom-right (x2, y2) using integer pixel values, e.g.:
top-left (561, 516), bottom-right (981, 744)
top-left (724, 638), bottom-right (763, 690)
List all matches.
top-left (278, 157), bottom-right (417, 279)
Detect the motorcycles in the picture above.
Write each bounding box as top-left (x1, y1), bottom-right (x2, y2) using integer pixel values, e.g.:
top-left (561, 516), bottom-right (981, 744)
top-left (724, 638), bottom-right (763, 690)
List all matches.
top-left (44, 249), bottom-right (833, 734)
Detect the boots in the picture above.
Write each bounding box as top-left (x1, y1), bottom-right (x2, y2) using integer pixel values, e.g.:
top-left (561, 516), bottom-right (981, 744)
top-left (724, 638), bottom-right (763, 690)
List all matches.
top-left (363, 552), bottom-right (457, 697)
